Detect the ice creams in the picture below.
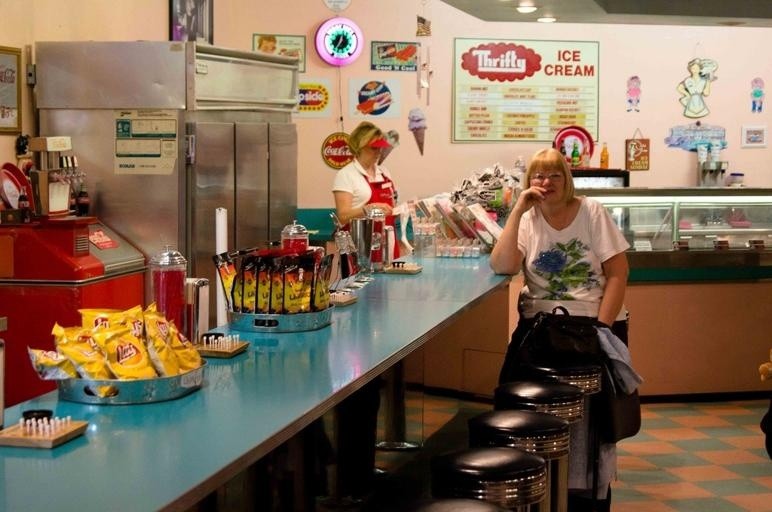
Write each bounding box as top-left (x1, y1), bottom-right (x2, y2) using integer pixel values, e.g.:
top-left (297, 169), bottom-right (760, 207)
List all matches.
top-left (408, 109), bottom-right (428, 155)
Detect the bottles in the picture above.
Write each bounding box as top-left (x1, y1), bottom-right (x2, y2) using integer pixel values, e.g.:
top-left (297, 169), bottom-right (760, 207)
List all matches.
top-left (17, 155), bottom-right (33, 173)
top-left (70, 182), bottom-right (89, 217)
top-left (151, 245), bottom-right (209, 345)
top-left (368, 208), bottom-right (387, 273)
top-left (17, 186), bottom-right (30, 223)
top-left (281, 220), bottom-right (308, 250)
top-left (552, 138), bottom-right (609, 169)
top-left (630, 143), bottom-right (635, 162)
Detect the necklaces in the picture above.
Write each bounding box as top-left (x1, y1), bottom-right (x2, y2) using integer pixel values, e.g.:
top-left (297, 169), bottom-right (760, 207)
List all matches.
top-left (547, 216), bottom-right (570, 228)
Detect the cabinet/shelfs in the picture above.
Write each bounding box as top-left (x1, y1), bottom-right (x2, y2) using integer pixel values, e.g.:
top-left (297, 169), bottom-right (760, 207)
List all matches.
top-left (404, 188), bottom-right (772, 403)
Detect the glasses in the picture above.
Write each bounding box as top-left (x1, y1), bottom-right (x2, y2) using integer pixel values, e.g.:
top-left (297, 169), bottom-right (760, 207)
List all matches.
top-left (529, 171), bottom-right (564, 184)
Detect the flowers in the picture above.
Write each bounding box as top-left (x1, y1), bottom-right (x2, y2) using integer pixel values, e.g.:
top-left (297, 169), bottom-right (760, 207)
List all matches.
top-left (529, 236), bottom-right (600, 301)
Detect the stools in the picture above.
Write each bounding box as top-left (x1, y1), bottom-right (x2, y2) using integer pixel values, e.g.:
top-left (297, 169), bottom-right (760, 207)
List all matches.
top-left (415, 362), bottom-right (602, 512)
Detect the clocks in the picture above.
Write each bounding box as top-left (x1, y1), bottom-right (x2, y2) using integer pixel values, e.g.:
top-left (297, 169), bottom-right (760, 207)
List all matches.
top-left (314, 17), bottom-right (363, 66)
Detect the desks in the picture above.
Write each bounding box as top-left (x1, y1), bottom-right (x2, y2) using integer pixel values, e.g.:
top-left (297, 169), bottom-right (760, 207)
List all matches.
top-left (0, 247), bottom-right (526, 511)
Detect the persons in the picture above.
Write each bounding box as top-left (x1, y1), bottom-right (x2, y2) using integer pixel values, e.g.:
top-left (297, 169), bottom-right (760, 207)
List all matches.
top-left (332, 122), bottom-right (401, 259)
top-left (490, 149), bottom-right (632, 512)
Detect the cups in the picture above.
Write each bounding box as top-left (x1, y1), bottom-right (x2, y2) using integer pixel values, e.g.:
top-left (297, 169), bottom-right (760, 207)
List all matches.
top-left (352, 217), bottom-right (371, 271)
top-left (727, 173), bottom-right (744, 185)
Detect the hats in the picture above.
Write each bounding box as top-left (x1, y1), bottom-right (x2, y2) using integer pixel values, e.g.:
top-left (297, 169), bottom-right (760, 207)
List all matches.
top-left (366, 138), bottom-right (392, 149)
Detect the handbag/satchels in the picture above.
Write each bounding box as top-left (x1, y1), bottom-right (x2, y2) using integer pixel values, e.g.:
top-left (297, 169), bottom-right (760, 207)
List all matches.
top-left (518, 305), bottom-right (599, 369)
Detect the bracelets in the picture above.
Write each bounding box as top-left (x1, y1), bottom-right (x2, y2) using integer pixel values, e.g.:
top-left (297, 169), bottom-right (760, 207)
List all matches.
top-left (362, 207), bottom-right (369, 218)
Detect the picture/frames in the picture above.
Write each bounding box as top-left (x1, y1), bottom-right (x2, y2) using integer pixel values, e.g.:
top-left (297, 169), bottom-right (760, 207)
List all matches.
top-left (742, 125), bottom-right (768, 149)
top-left (0, 45), bottom-right (22, 135)
top-left (169, 1), bottom-right (214, 44)
